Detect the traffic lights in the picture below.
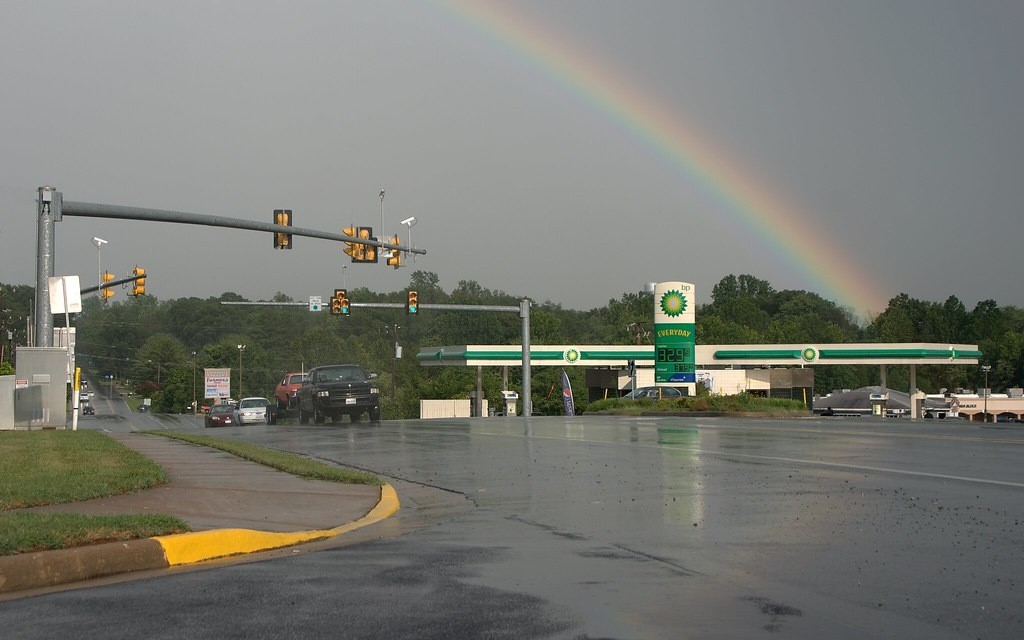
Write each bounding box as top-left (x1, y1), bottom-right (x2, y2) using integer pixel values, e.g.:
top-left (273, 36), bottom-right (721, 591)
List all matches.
top-left (343, 228), bottom-right (355, 257)
top-left (104, 270), bottom-right (115, 298)
top-left (329, 289), bottom-right (350, 316)
top-left (387, 237), bottom-right (400, 269)
top-left (133, 268), bottom-right (145, 296)
top-left (408, 291), bottom-right (418, 313)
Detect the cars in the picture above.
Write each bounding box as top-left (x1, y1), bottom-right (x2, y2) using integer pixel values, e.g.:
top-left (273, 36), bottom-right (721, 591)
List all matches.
top-left (87, 391), bottom-right (94, 397)
top-left (80, 393), bottom-right (89, 402)
top-left (82, 407), bottom-right (95, 415)
top-left (137, 405), bottom-right (152, 412)
top-left (273, 372), bottom-right (311, 408)
top-left (81, 381), bottom-right (88, 388)
top-left (296, 364), bottom-right (380, 425)
top-left (233, 398), bottom-right (271, 426)
top-left (624, 386), bottom-right (687, 400)
top-left (204, 404), bottom-right (236, 427)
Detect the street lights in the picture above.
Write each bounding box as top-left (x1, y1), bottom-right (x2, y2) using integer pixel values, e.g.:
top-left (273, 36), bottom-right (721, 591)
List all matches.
top-left (192, 351), bottom-right (197, 414)
top-left (238, 345), bottom-right (246, 398)
top-left (981, 365), bottom-right (991, 423)
